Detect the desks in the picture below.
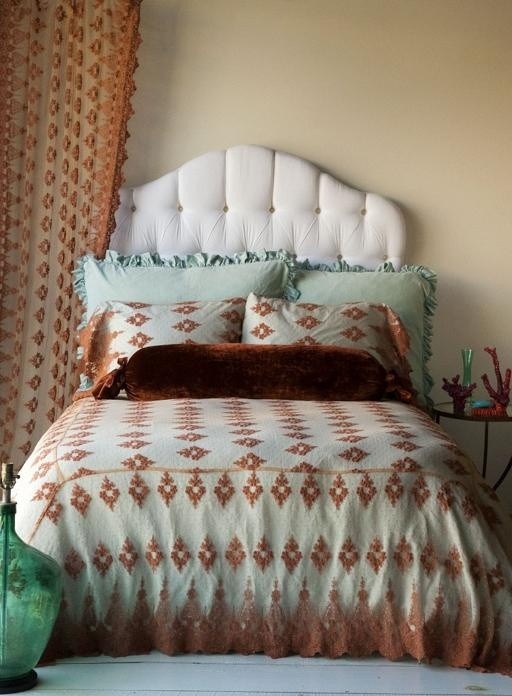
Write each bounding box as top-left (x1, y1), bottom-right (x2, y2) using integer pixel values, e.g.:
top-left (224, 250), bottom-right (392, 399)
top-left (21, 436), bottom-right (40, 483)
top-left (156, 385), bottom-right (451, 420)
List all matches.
top-left (435, 396), bottom-right (512, 500)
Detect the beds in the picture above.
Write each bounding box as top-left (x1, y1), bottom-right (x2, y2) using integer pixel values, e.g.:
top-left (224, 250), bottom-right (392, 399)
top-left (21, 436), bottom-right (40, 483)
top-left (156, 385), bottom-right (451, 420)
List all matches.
top-left (12, 145), bottom-right (508, 690)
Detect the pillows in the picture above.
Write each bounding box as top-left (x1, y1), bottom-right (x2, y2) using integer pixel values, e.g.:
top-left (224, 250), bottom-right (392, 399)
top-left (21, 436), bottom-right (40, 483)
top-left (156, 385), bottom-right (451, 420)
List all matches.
top-left (71, 249), bottom-right (300, 388)
top-left (242, 260), bottom-right (436, 408)
top-left (91, 343), bottom-right (411, 401)
